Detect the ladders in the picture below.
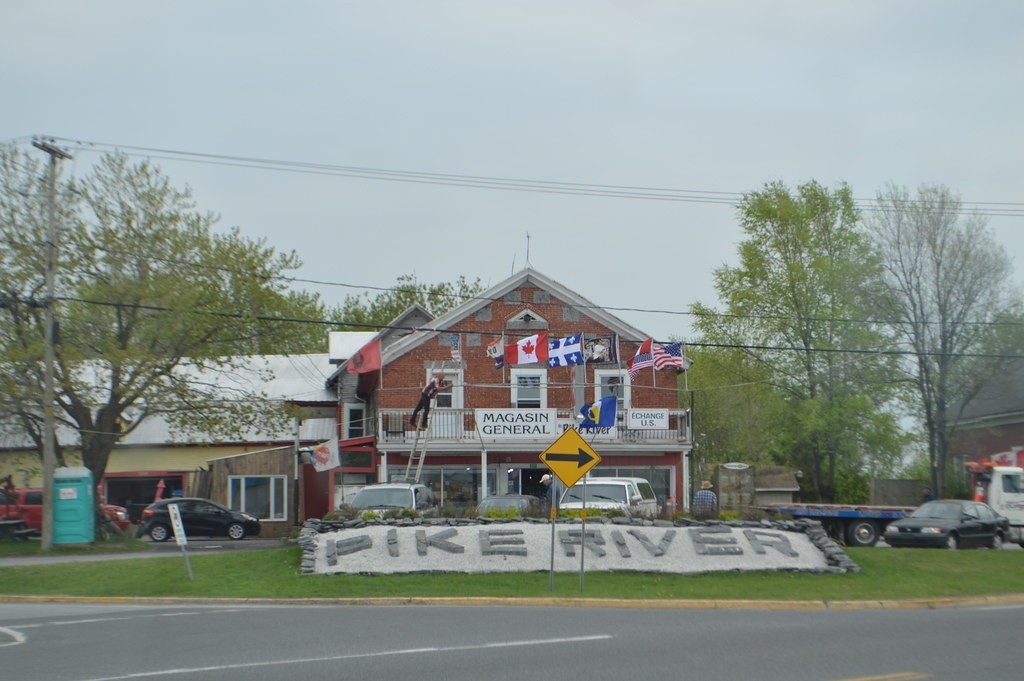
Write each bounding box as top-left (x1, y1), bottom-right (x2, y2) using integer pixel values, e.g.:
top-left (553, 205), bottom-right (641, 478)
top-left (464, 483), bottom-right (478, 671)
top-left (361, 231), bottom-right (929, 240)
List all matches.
top-left (403, 361), bottom-right (446, 483)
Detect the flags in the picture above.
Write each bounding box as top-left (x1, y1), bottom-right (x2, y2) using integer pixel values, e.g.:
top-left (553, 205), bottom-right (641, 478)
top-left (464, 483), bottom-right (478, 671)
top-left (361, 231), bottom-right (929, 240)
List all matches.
top-left (347, 341), bottom-right (380, 373)
top-left (627, 338), bottom-right (652, 380)
top-left (653, 341), bottom-right (683, 370)
top-left (990, 448), bottom-right (1024, 468)
top-left (311, 438), bottom-right (340, 472)
top-left (507, 330), bottom-right (549, 365)
top-left (581, 396), bottom-right (617, 428)
top-left (549, 332), bottom-right (584, 367)
top-left (584, 334), bottom-right (618, 363)
top-left (486, 336), bottom-right (504, 368)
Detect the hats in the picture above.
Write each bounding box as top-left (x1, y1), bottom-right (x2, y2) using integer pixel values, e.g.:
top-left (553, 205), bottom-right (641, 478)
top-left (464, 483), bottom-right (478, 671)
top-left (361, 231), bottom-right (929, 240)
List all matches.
top-left (701, 481), bottom-right (713, 490)
top-left (538, 474), bottom-right (553, 483)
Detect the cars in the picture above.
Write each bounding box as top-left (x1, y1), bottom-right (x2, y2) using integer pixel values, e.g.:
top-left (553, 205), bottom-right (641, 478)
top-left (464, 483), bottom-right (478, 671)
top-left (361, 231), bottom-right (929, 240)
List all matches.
top-left (559, 476), bottom-right (659, 518)
top-left (885, 499), bottom-right (1009, 550)
top-left (137, 496), bottom-right (261, 542)
top-left (476, 495), bottom-right (540, 519)
top-left (0, 488), bottom-right (130, 538)
top-left (340, 482), bottom-right (440, 520)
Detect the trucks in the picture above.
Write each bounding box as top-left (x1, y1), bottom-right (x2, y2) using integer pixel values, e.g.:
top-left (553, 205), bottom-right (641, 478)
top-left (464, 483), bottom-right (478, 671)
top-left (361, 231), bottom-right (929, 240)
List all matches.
top-left (767, 466), bottom-right (1024, 548)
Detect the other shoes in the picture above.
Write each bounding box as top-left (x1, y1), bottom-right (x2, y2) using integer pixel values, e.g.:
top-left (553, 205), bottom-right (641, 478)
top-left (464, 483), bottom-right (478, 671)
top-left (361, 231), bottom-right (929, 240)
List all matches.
top-left (409, 422), bottom-right (427, 431)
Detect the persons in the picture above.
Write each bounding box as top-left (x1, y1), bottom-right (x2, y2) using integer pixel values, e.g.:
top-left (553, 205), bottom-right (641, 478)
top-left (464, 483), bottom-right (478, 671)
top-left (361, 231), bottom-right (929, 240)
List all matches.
top-left (693, 481), bottom-right (717, 517)
top-left (539, 474), bottom-right (559, 505)
top-left (409, 372), bottom-right (453, 428)
top-left (921, 487), bottom-right (935, 501)
